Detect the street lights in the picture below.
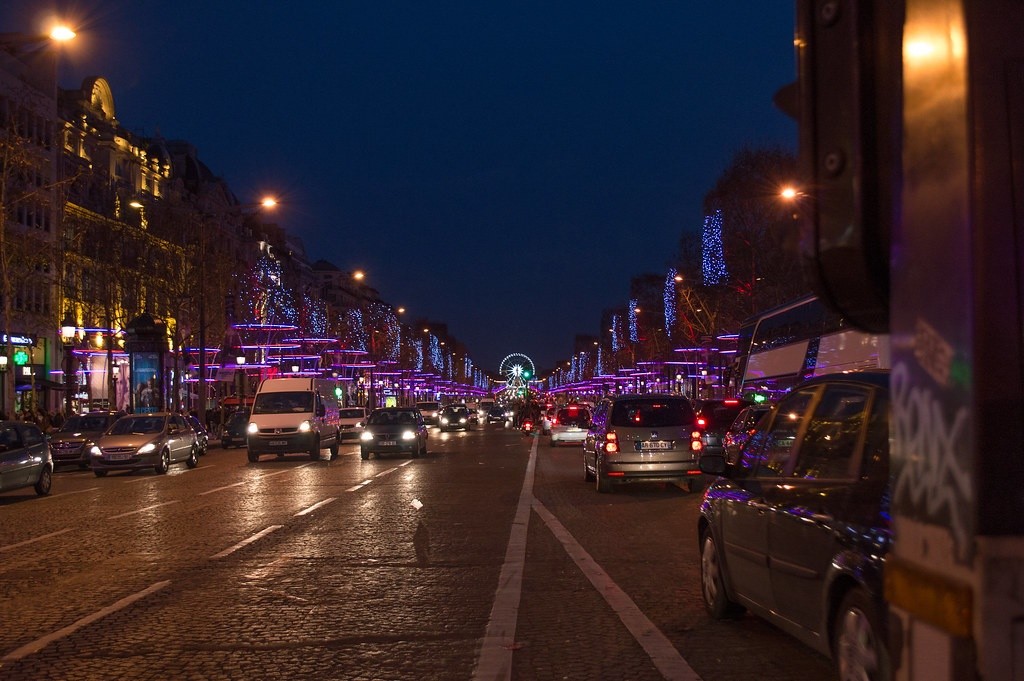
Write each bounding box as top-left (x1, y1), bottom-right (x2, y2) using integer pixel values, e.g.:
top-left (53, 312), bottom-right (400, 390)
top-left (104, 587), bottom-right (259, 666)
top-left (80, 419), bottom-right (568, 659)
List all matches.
top-left (44, 25), bottom-right (78, 409)
top-left (198, 198), bottom-right (279, 428)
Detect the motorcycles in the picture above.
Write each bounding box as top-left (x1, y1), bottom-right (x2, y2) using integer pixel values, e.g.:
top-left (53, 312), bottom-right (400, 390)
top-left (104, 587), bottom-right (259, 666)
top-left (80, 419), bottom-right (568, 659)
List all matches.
top-left (520, 417), bottom-right (535, 437)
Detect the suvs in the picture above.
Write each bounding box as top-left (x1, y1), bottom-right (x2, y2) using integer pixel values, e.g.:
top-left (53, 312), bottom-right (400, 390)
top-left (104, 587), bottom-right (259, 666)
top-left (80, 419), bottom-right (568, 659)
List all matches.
top-left (337, 407), bottom-right (372, 440)
top-left (487, 406), bottom-right (507, 425)
top-left (415, 401), bottom-right (441, 427)
top-left (439, 403), bottom-right (472, 433)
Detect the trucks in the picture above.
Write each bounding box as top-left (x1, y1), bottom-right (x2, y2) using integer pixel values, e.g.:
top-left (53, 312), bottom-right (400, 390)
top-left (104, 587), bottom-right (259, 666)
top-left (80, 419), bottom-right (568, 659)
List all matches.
top-left (245, 376), bottom-right (343, 464)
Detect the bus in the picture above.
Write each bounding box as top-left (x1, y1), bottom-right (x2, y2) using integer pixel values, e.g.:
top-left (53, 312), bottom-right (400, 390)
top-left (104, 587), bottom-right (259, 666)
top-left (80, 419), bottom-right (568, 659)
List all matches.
top-left (734, 293), bottom-right (909, 404)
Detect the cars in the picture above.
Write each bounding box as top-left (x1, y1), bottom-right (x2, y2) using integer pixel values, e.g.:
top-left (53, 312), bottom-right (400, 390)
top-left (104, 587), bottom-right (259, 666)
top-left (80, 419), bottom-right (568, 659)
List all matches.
top-left (542, 405), bottom-right (593, 447)
top-left (48, 408), bottom-right (209, 478)
top-left (360, 407), bottom-right (429, 460)
top-left (584, 391), bottom-right (704, 495)
top-left (0, 419), bottom-right (55, 497)
top-left (221, 411), bottom-right (253, 451)
top-left (693, 373), bottom-right (899, 679)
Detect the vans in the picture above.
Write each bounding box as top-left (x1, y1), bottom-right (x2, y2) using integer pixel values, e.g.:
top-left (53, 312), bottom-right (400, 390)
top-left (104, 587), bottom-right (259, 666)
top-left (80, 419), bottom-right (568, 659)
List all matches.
top-left (479, 398), bottom-right (495, 418)
